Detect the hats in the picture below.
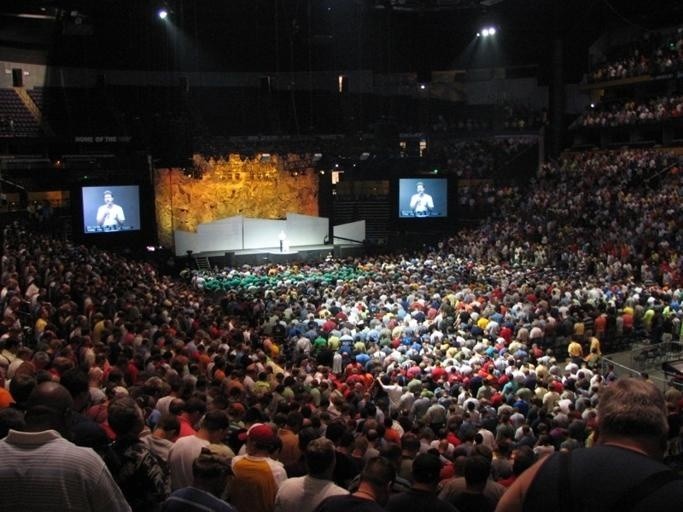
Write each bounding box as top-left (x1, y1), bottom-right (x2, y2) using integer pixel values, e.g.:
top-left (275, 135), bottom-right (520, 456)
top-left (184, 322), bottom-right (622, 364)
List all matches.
top-left (236, 423), bottom-right (276, 443)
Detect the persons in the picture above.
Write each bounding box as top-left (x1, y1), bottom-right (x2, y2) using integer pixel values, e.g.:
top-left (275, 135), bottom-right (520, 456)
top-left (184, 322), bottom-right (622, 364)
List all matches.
top-left (97, 190), bottom-right (126, 231)
top-left (400, 238), bottom-right (453, 353)
top-left (395, 353), bottom-right (682, 512)
top-left (411, 182), bottom-right (434, 217)
top-left (0, 209), bottom-right (400, 512)
top-left (451, 26), bottom-right (683, 351)
